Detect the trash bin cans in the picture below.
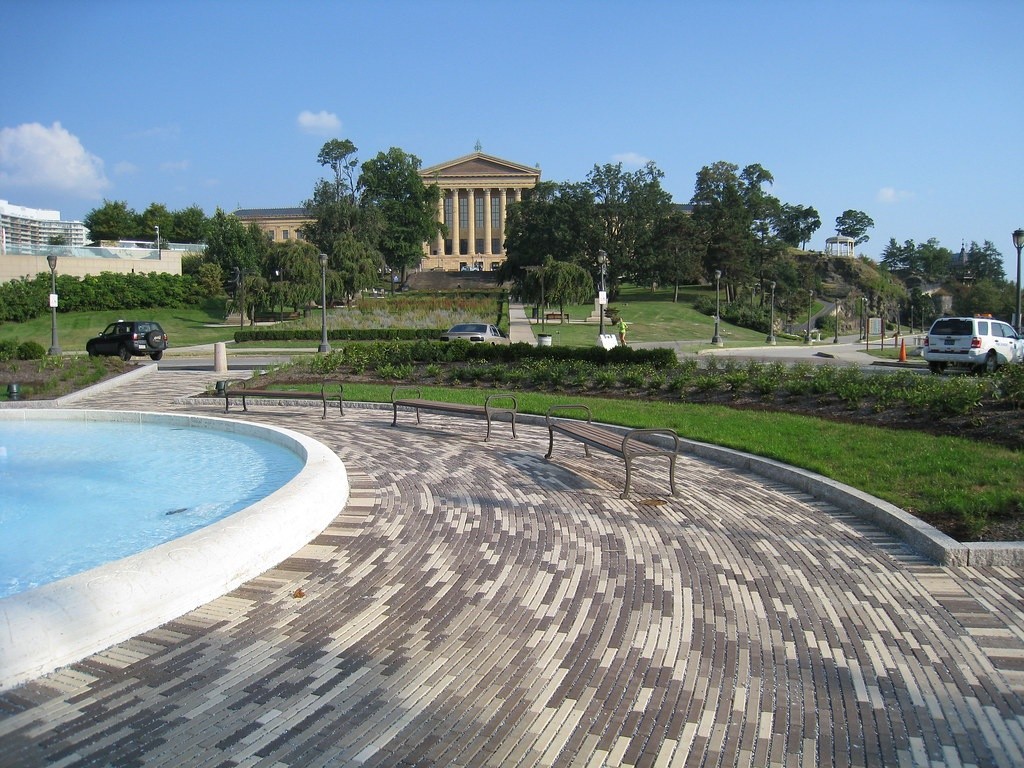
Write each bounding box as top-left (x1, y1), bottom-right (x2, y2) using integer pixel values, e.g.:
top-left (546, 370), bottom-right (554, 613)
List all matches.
top-left (532, 308), bottom-right (538, 319)
top-left (538, 333), bottom-right (552, 347)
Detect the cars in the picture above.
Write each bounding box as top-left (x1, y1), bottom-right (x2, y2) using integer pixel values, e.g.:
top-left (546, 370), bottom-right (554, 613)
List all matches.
top-left (440, 322), bottom-right (511, 347)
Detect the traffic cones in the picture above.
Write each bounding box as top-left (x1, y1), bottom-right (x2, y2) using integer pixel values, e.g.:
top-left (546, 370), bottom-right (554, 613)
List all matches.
top-left (897, 338), bottom-right (907, 362)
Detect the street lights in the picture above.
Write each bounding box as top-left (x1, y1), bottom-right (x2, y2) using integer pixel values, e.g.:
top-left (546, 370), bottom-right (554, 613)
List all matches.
top-left (712, 269), bottom-right (723, 346)
top-left (804, 289), bottom-right (812, 345)
top-left (862, 301), bottom-right (869, 339)
top-left (833, 298), bottom-right (839, 344)
top-left (46, 252), bottom-right (61, 355)
top-left (910, 305), bottom-right (913, 334)
top-left (1011, 228), bottom-right (1024, 335)
top-left (154, 225), bottom-right (159, 256)
top-left (595, 247), bottom-right (606, 335)
top-left (921, 307), bottom-right (924, 333)
top-left (766, 280), bottom-right (776, 345)
top-left (318, 252), bottom-right (331, 352)
top-left (897, 304), bottom-right (902, 335)
top-left (276, 267), bottom-right (283, 323)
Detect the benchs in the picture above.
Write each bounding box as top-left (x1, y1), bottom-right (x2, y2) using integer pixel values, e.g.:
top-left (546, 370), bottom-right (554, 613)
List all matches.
top-left (544, 405), bottom-right (682, 497)
top-left (546, 314), bottom-right (569, 323)
top-left (390, 384), bottom-right (519, 442)
top-left (224, 378), bottom-right (346, 420)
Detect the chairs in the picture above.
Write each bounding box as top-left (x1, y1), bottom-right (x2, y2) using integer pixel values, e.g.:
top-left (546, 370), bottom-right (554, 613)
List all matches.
top-left (119, 327), bottom-right (126, 333)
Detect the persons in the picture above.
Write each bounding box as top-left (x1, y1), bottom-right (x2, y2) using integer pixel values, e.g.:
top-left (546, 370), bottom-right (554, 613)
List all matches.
top-left (615, 317), bottom-right (628, 346)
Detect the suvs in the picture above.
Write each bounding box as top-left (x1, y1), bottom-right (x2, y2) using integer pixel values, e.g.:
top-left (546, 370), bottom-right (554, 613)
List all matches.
top-left (86, 321), bottom-right (169, 361)
top-left (921, 313), bottom-right (1024, 376)
